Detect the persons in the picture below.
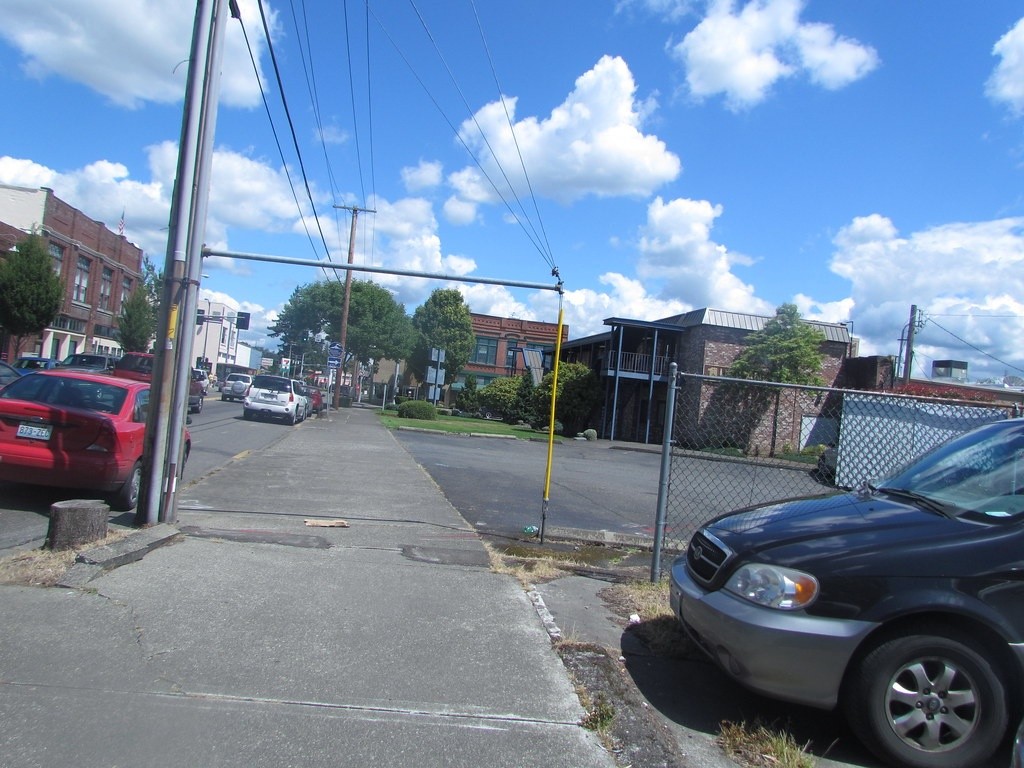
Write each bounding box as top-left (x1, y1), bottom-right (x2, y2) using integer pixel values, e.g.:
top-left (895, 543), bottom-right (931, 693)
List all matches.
top-left (208, 373), bottom-right (218, 388)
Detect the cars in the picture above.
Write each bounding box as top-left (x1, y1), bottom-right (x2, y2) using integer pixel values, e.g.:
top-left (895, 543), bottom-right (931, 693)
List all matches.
top-left (300, 381), bottom-right (333, 418)
top-left (11, 358), bottom-right (60, 376)
top-left (0, 361), bottom-right (192, 511)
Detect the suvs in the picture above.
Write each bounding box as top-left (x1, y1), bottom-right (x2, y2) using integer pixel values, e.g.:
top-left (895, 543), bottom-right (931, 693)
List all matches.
top-left (244, 375), bottom-right (308, 426)
top-left (193, 369), bottom-right (209, 396)
top-left (113, 352), bottom-right (203, 414)
top-left (56, 352), bottom-right (122, 373)
top-left (221, 372), bottom-right (252, 402)
top-left (670, 418), bottom-right (1023, 767)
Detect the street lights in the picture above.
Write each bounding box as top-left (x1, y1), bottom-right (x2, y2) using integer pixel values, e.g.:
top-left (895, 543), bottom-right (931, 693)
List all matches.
top-left (202, 298), bottom-right (210, 364)
